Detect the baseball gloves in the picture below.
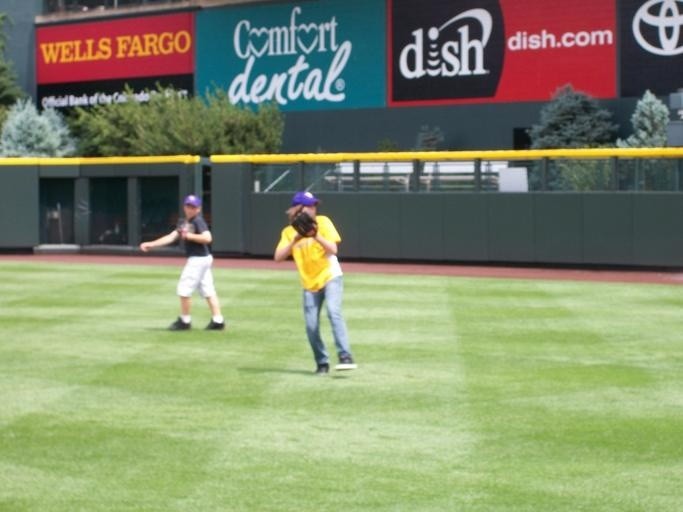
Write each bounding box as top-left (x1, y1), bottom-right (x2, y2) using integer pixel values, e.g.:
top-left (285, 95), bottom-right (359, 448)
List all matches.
top-left (175, 216), bottom-right (195, 239)
top-left (291, 211), bottom-right (317, 237)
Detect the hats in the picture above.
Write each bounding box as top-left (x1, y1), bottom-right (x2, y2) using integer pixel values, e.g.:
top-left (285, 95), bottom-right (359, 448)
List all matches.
top-left (292, 191), bottom-right (320, 206)
top-left (185, 195), bottom-right (200, 207)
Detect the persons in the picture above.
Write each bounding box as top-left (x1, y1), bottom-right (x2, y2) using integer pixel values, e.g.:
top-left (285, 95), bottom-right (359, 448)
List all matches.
top-left (139, 195), bottom-right (224, 331)
top-left (272, 190), bottom-right (357, 374)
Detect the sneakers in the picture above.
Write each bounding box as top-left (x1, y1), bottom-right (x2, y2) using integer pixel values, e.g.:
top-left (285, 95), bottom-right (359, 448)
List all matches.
top-left (206, 319), bottom-right (224, 329)
top-left (338, 350), bottom-right (352, 364)
top-left (315, 364), bottom-right (329, 376)
top-left (169, 317), bottom-right (191, 330)
